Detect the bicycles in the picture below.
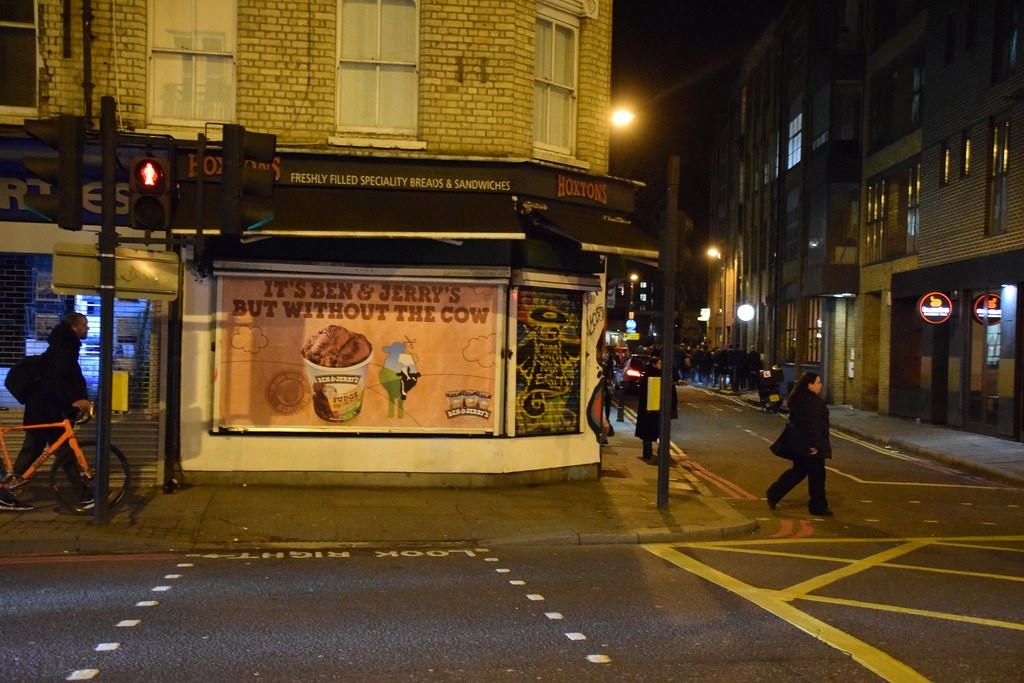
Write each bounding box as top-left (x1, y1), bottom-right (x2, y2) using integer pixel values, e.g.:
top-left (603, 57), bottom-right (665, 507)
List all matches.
top-left (0, 402), bottom-right (130, 515)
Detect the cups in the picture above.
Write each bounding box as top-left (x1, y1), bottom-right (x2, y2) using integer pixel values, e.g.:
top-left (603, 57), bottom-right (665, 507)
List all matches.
top-left (299, 349), bottom-right (375, 423)
top-left (463, 388), bottom-right (479, 408)
top-left (477, 392), bottom-right (492, 410)
top-left (445, 390), bottom-right (464, 409)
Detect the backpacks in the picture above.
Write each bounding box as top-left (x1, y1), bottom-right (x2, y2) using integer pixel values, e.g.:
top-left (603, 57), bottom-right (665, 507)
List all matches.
top-left (5, 342), bottom-right (56, 405)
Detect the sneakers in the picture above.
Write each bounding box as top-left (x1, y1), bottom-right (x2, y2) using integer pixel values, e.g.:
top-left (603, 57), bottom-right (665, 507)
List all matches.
top-left (80, 485), bottom-right (116, 510)
top-left (0, 490), bottom-right (34, 510)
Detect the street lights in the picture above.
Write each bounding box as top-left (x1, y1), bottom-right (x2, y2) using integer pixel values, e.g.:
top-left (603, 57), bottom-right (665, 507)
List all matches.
top-left (709, 249), bottom-right (727, 348)
top-left (611, 109), bottom-right (682, 507)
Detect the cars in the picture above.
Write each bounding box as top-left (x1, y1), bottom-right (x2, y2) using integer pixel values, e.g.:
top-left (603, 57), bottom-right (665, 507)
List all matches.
top-left (614, 348), bottom-right (629, 374)
top-left (615, 356), bottom-right (652, 392)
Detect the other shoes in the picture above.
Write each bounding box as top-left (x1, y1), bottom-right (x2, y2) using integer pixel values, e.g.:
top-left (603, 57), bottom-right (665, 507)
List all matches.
top-left (810, 509), bottom-right (835, 517)
top-left (766, 491), bottom-right (777, 509)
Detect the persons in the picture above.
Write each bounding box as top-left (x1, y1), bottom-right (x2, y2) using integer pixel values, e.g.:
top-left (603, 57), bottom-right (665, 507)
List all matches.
top-left (0, 313), bottom-right (115, 511)
top-left (635, 357), bottom-right (678, 458)
top-left (674, 342), bottom-right (761, 392)
top-left (766, 372), bottom-right (834, 516)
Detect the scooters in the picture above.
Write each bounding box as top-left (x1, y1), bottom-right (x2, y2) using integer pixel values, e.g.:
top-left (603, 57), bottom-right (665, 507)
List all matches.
top-left (749, 365), bottom-right (783, 414)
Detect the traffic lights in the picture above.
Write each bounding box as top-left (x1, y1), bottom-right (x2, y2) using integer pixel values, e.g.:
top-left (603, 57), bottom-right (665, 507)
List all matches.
top-left (23, 114), bottom-right (84, 231)
top-left (220, 124), bottom-right (277, 236)
top-left (128, 156), bottom-right (172, 230)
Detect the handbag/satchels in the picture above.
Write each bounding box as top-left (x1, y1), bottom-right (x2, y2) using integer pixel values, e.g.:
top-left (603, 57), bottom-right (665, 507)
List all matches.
top-left (770, 423), bottom-right (797, 461)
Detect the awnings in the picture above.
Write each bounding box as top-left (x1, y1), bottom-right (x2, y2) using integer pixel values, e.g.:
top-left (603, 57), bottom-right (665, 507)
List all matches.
top-left (522, 198), bottom-right (660, 267)
top-left (169, 181), bottom-right (527, 241)
